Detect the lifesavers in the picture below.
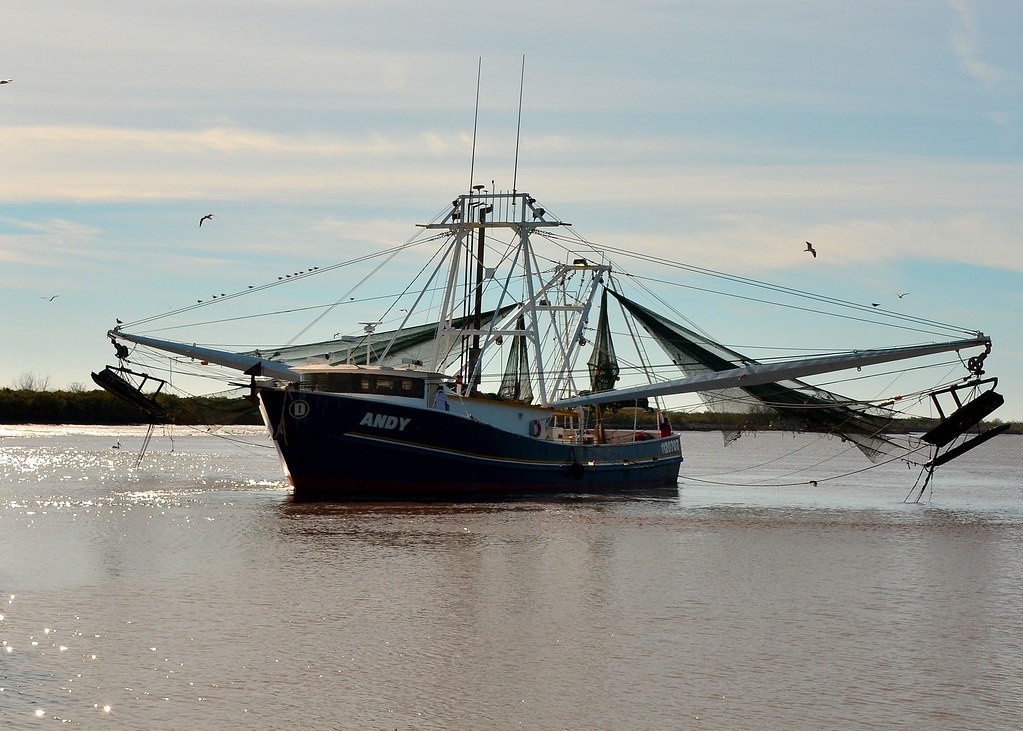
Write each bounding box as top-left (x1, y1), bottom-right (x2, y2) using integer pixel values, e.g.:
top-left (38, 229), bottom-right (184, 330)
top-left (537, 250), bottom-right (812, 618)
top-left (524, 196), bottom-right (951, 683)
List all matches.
top-left (529, 420), bottom-right (541, 438)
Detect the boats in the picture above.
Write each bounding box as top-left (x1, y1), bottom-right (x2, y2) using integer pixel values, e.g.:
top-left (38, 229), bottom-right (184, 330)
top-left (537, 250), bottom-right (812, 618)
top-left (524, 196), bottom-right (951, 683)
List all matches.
top-left (104, 50), bottom-right (1008, 505)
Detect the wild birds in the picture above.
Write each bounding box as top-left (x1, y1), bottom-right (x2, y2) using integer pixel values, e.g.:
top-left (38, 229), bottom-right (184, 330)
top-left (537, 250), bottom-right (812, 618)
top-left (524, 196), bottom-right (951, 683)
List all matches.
top-left (895, 292), bottom-right (909, 300)
top-left (804, 241), bottom-right (817, 257)
top-left (199, 213), bottom-right (214, 228)
top-left (40, 293), bottom-right (59, 301)
